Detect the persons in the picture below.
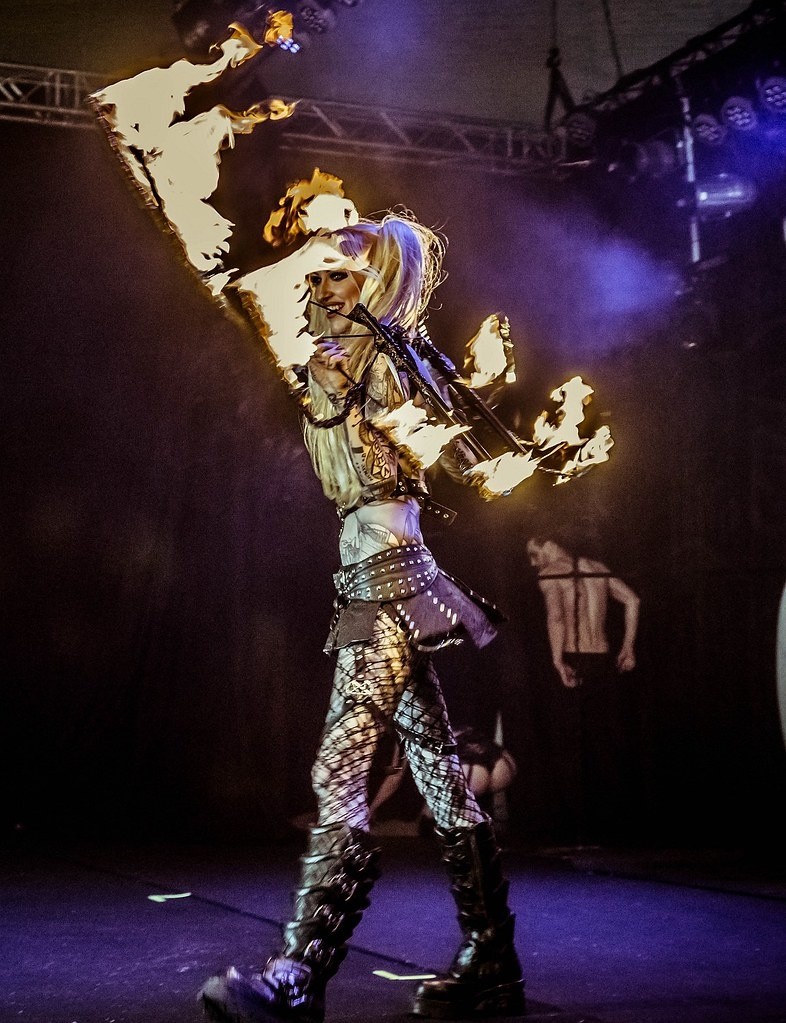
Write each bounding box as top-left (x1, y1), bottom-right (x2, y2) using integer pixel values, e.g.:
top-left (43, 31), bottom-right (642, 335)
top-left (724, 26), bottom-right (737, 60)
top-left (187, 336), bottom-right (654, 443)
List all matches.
top-left (525, 533), bottom-right (641, 837)
top-left (366, 713), bottom-right (519, 845)
top-left (199, 198), bottom-right (526, 1023)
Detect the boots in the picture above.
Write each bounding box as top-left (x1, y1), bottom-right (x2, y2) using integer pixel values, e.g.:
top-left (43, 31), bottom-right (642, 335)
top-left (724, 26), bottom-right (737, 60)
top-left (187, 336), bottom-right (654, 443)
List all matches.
top-left (410, 810), bottom-right (531, 1015)
top-left (198, 821), bottom-right (383, 1023)
